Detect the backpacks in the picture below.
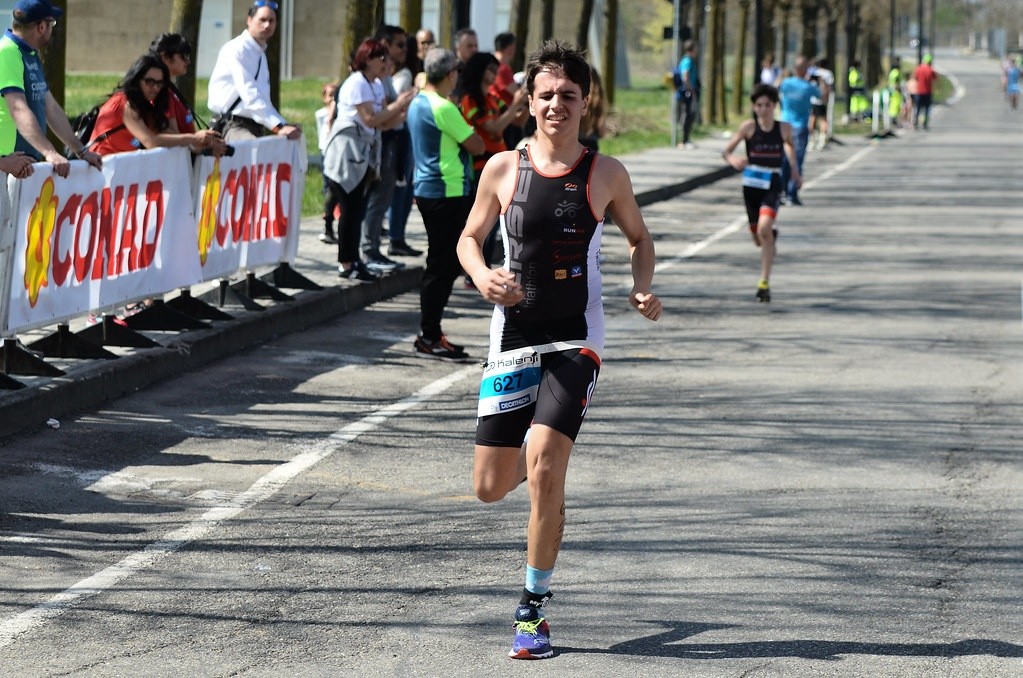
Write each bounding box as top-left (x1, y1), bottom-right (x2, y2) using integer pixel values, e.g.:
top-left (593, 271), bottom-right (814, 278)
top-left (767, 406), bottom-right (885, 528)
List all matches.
top-left (65, 100), bottom-right (126, 159)
top-left (673, 65), bottom-right (684, 93)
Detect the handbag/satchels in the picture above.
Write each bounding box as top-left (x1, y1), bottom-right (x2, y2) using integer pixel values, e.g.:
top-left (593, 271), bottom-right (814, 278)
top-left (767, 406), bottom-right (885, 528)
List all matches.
top-left (209, 113), bottom-right (231, 137)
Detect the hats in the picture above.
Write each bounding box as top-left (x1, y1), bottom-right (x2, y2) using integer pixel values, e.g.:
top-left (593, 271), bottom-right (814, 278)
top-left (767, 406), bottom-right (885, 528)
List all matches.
top-left (922, 53), bottom-right (934, 64)
top-left (425, 48), bottom-right (460, 76)
top-left (13, 0), bottom-right (63, 23)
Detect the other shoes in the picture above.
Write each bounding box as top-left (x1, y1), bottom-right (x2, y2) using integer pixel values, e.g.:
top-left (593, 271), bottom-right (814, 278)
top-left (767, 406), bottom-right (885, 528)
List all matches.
top-left (388, 240), bottom-right (423, 256)
top-left (123, 301), bottom-right (147, 318)
top-left (380, 228), bottom-right (390, 238)
top-left (363, 251), bottom-right (406, 273)
top-left (465, 278), bottom-right (478, 290)
top-left (319, 234), bottom-right (338, 243)
top-left (756, 282), bottom-right (771, 303)
top-left (87, 313), bottom-right (128, 329)
top-left (338, 264), bottom-right (383, 284)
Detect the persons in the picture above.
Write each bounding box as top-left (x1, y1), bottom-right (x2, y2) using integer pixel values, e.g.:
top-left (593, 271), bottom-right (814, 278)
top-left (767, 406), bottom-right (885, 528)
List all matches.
top-left (721, 82), bottom-right (803, 302)
top-left (207, 0), bottom-right (303, 289)
top-left (673, 39), bottom-right (940, 207)
top-left (86, 32), bottom-right (227, 330)
top-left (314, 24), bottom-right (603, 281)
top-left (1001, 56), bottom-right (1023, 110)
top-left (0, 0), bottom-right (103, 178)
top-left (406, 45), bottom-right (485, 363)
top-left (455, 39), bottom-right (664, 659)
top-left (0, 150), bottom-right (38, 179)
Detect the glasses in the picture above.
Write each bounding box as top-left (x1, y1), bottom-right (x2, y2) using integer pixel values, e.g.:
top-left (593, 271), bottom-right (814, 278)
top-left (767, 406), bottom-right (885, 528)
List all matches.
top-left (42, 16), bottom-right (57, 26)
top-left (143, 77), bottom-right (166, 89)
top-left (397, 41), bottom-right (407, 48)
top-left (254, 0), bottom-right (279, 9)
top-left (421, 39), bottom-right (435, 44)
top-left (380, 56), bottom-right (387, 62)
top-left (183, 52), bottom-right (191, 61)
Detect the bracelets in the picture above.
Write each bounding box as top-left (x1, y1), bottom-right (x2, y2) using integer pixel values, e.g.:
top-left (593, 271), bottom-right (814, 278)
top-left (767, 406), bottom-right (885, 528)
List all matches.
top-left (75, 145), bottom-right (89, 159)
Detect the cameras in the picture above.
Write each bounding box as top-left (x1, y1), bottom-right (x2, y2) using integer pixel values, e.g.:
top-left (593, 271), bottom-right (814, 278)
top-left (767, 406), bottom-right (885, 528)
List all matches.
top-left (203, 95), bottom-right (243, 157)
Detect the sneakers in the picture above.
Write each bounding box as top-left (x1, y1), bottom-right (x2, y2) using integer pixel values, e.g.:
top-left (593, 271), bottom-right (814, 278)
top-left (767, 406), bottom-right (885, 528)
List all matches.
top-left (509, 614), bottom-right (553, 660)
top-left (413, 333), bottom-right (470, 363)
